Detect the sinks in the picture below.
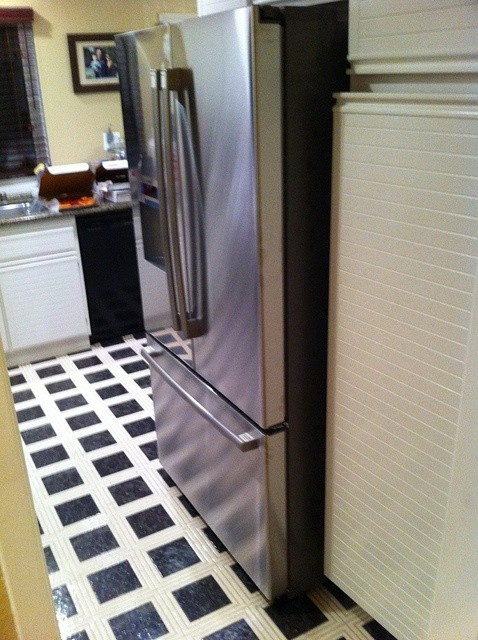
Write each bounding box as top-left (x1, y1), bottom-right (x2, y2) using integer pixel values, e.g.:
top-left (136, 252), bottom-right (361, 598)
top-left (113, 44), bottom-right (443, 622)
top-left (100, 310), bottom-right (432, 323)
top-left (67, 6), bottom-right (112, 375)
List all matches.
top-left (0, 203), bottom-right (47, 218)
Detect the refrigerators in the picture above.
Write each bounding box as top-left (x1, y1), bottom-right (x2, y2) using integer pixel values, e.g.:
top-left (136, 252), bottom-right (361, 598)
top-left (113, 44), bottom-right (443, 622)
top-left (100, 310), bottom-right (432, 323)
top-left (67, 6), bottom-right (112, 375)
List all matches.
top-left (114, 4), bottom-right (348, 602)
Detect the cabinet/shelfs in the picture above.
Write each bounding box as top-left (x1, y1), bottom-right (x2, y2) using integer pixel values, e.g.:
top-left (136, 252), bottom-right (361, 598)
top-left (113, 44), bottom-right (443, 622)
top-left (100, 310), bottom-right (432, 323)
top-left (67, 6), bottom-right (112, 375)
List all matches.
top-left (0, 215), bottom-right (91, 371)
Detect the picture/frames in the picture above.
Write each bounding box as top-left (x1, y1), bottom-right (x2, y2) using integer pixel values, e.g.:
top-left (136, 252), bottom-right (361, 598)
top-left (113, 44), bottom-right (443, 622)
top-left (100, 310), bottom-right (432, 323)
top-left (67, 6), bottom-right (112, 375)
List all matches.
top-left (66, 32), bottom-right (122, 94)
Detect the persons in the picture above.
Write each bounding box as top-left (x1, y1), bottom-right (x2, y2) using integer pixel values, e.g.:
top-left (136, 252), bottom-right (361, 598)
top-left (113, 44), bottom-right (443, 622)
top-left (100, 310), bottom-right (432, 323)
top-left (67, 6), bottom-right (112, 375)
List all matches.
top-left (90, 48), bottom-right (115, 76)
